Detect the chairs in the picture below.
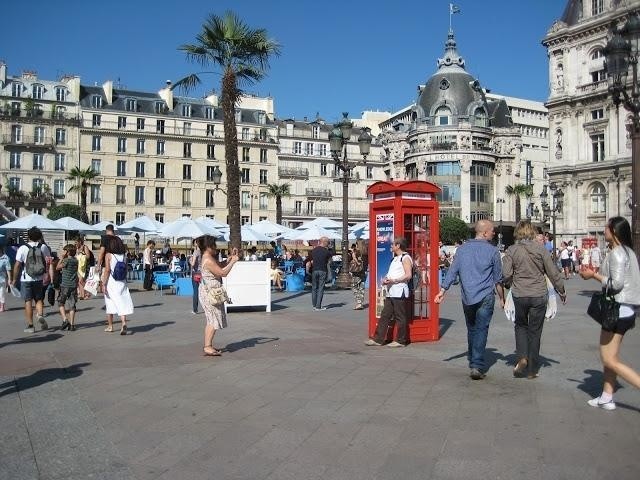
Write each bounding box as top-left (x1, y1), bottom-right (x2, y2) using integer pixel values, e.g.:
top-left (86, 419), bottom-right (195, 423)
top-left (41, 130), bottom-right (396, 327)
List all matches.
top-left (153, 271), bottom-right (176, 297)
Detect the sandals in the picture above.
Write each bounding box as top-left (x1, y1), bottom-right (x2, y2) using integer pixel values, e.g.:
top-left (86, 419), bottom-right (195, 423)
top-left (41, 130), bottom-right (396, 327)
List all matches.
top-left (353, 306), bottom-right (364, 310)
top-left (312, 306), bottom-right (315, 310)
top-left (203, 347), bottom-right (221, 356)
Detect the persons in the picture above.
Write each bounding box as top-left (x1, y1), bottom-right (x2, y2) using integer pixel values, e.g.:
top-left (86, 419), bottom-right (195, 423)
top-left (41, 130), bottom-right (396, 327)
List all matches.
top-left (12, 228), bottom-right (54, 332)
top-left (103, 236), bottom-right (134, 334)
top-left (438, 239), bottom-right (453, 272)
top-left (245, 249), bottom-right (258, 261)
top-left (578, 217), bottom-right (640, 409)
top-left (134, 233), bottom-right (140, 253)
top-left (505, 226), bottom-right (558, 323)
top-left (143, 240), bottom-right (157, 289)
top-left (591, 243), bottom-right (601, 271)
top-left (311, 237), bottom-right (332, 311)
top-left (364, 237), bottom-right (413, 347)
top-left (289, 249), bottom-right (294, 260)
top-left (349, 239), bottom-right (368, 310)
top-left (162, 243), bottom-right (169, 255)
top-left (568, 240), bottom-right (576, 273)
top-left (582, 244), bottom-right (590, 271)
top-left (74, 233), bottom-right (90, 300)
top-left (198, 235), bottom-right (240, 355)
top-left (270, 242), bottom-right (281, 255)
top-left (294, 249), bottom-right (302, 260)
top-left (304, 251), bottom-right (313, 265)
top-left (179, 254), bottom-right (186, 266)
top-left (502, 220), bottom-right (566, 380)
top-left (97, 224), bottom-right (116, 281)
top-left (304, 259), bottom-right (313, 281)
top-left (433, 220), bottom-right (505, 380)
top-left (575, 246), bottom-right (580, 266)
top-left (561, 242), bottom-right (570, 281)
top-left (6, 239), bottom-right (17, 264)
top-left (282, 244), bottom-right (288, 256)
top-left (189, 238), bottom-right (201, 314)
top-left (272, 261), bottom-right (284, 292)
top-left (56, 244), bottom-right (79, 331)
top-left (127, 253), bottom-right (131, 258)
top-left (0, 245), bottom-right (13, 312)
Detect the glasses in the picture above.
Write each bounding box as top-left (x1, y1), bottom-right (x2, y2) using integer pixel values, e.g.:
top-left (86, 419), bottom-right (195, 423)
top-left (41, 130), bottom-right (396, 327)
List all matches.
top-left (392, 243), bottom-right (399, 246)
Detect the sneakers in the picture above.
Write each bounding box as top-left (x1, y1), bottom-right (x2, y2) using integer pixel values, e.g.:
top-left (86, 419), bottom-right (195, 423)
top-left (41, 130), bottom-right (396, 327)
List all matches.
top-left (104, 326), bottom-right (114, 332)
top-left (527, 372), bottom-right (540, 379)
top-left (68, 325), bottom-right (74, 331)
top-left (364, 339), bottom-right (382, 345)
top-left (24, 326), bottom-right (35, 333)
top-left (120, 325), bottom-right (127, 335)
top-left (61, 319), bottom-right (69, 330)
top-left (513, 358), bottom-right (527, 377)
top-left (37, 316), bottom-right (48, 330)
top-left (315, 307), bottom-right (326, 311)
top-left (192, 311), bottom-right (197, 314)
top-left (471, 367), bottom-right (481, 380)
top-left (587, 396), bottom-right (616, 411)
top-left (387, 340), bottom-right (406, 347)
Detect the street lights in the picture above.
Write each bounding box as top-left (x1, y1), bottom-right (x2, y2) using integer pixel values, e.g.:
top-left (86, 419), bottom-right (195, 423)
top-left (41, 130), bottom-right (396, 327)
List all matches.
top-left (543, 184), bottom-right (564, 273)
top-left (212, 166), bottom-right (228, 195)
top-left (534, 189), bottom-right (550, 237)
top-left (328, 112), bottom-right (371, 290)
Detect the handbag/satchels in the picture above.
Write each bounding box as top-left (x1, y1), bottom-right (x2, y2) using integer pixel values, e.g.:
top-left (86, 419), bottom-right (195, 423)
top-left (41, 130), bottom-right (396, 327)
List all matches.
top-left (194, 274), bottom-right (201, 282)
top-left (350, 259), bottom-right (363, 273)
top-left (48, 287), bottom-right (55, 306)
top-left (208, 286), bottom-right (228, 306)
top-left (587, 278), bottom-right (619, 331)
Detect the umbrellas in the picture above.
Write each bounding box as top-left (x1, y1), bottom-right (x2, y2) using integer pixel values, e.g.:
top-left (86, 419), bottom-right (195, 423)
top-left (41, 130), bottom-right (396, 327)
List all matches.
top-left (226, 226), bottom-right (274, 243)
top-left (251, 220), bottom-right (297, 234)
top-left (197, 215), bottom-right (227, 228)
top-left (118, 216), bottom-right (167, 250)
top-left (159, 216), bottom-right (221, 256)
top-left (297, 216), bottom-right (343, 228)
top-left (349, 220), bottom-right (425, 233)
top-left (286, 223), bottom-right (342, 241)
top-left (0, 213), bottom-right (66, 229)
top-left (54, 216), bottom-right (98, 244)
top-left (92, 220), bottom-right (131, 235)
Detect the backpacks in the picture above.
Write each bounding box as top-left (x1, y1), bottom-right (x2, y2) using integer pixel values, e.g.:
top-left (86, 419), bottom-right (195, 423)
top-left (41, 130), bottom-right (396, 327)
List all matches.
top-left (23, 242), bottom-right (46, 276)
top-left (110, 253), bottom-right (127, 280)
